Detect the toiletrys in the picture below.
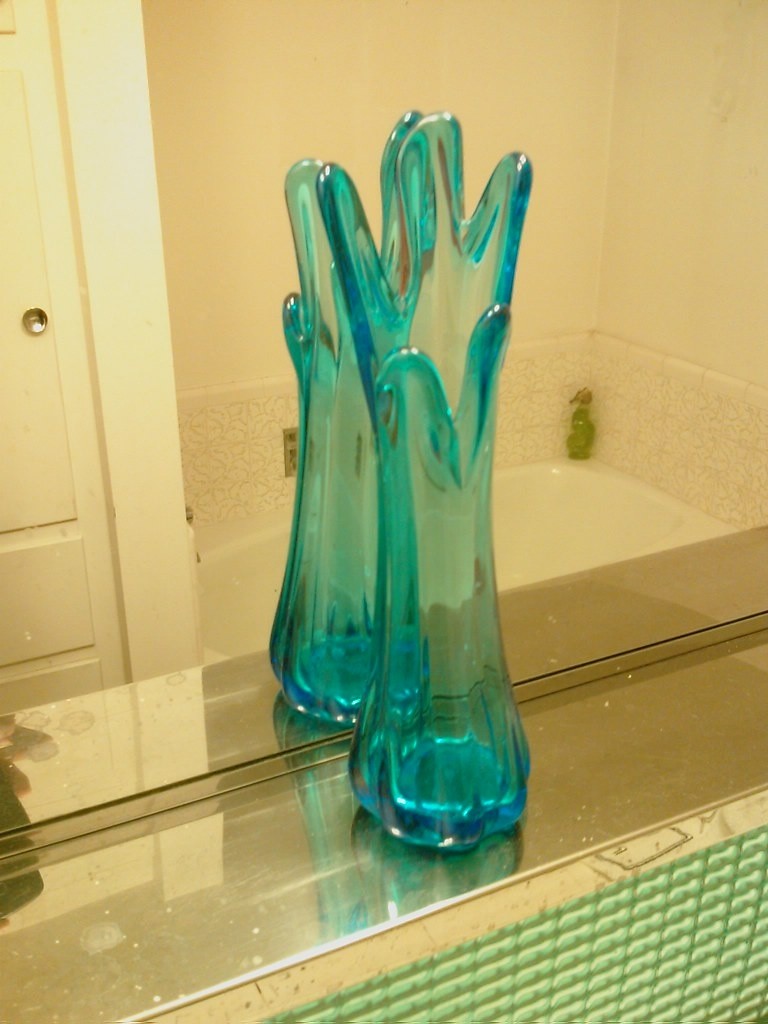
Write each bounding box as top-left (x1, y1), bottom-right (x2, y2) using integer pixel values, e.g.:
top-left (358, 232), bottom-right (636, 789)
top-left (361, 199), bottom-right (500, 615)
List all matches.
top-left (566, 387), bottom-right (595, 460)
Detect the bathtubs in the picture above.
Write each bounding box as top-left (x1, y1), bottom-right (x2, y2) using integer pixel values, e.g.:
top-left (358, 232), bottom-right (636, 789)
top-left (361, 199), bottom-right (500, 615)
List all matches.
top-left (201, 456), bottom-right (738, 667)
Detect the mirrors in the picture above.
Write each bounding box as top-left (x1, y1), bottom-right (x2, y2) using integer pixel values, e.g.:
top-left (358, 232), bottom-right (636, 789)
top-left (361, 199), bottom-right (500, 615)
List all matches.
top-left (0, 0), bottom-right (768, 860)
